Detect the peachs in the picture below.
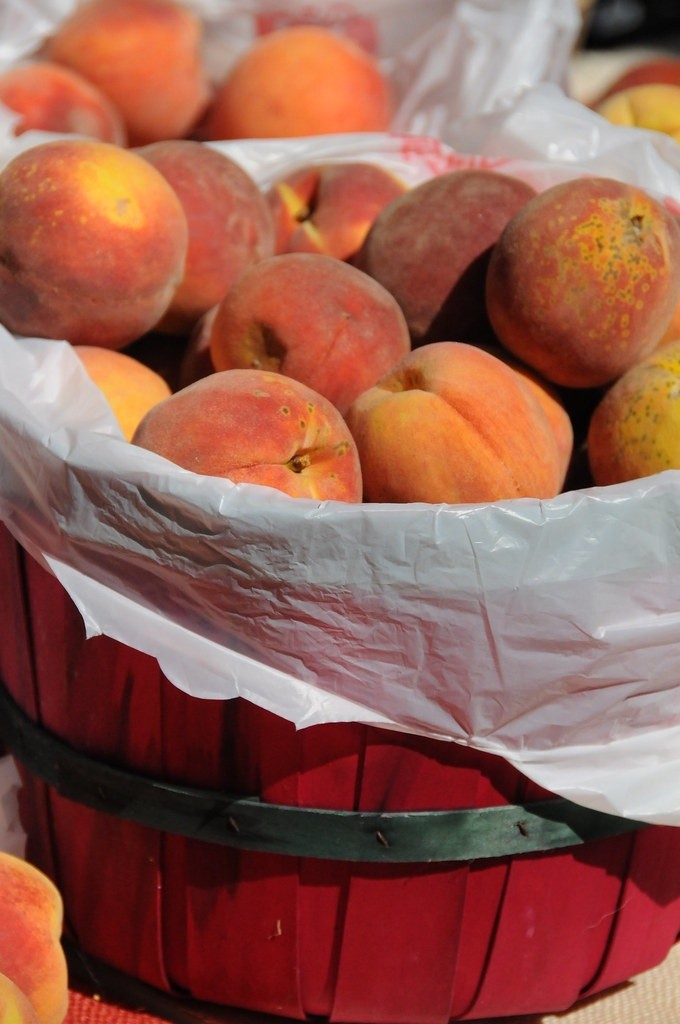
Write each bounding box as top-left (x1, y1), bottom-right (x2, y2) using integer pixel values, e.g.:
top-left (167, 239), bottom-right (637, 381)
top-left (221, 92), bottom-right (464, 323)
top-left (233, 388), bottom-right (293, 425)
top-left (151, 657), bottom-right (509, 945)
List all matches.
top-left (0, 849), bottom-right (68, 1024)
top-left (0, 0), bottom-right (680, 503)
